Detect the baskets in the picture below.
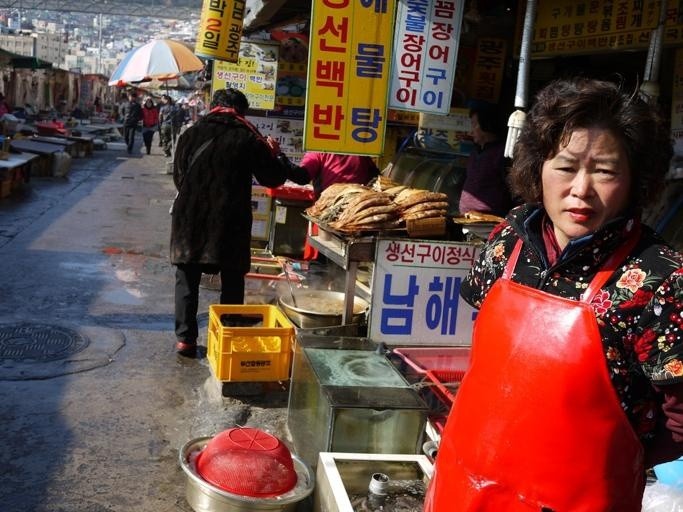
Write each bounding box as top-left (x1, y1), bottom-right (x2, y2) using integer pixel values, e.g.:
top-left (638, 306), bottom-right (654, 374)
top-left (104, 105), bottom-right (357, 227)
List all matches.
top-left (424, 369), bottom-right (466, 434)
top-left (195, 428), bottom-right (297, 497)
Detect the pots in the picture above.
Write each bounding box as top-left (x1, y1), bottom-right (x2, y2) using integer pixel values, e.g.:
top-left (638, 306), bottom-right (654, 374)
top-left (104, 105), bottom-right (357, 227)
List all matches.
top-left (277, 290), bottom-right (369, 328)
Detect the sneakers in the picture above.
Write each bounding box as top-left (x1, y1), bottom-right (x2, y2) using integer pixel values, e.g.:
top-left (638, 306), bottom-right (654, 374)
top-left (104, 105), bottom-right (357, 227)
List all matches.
top-left (176, 342), bottom-right (197, 355)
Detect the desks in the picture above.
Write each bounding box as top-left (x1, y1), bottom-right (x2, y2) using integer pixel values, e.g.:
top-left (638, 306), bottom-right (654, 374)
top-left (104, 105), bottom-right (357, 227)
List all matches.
top-left (266, 198), bottom-right (314, 254)
top-left (300, 213), bottom-right (376, 324)
top-left (0, 136), bottom-right (94, 199)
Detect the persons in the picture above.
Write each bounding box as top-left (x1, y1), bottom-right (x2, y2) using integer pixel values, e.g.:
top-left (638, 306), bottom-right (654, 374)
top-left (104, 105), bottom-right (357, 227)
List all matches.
top-left (424, 74), bottom-right (683, 512)
top-left (459, 101), bottom-right (516, 244)
top-left (117, 88), bottom-right (188, 156)
top-left (279, 152), bottom-right (385, 265)
top-left (169, 87), bottom-right (287, 358)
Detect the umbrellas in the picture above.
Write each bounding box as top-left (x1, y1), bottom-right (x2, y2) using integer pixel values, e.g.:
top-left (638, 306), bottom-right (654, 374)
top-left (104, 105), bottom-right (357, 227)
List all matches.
top-left (108, 36), bottom-right (205, 98)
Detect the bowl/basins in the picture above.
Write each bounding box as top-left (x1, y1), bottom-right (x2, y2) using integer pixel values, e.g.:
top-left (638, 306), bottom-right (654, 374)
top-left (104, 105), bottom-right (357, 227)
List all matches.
top-left (177, 434), bottom-right (315, 512)
top-left (289, 85), bottom-right (303, 97)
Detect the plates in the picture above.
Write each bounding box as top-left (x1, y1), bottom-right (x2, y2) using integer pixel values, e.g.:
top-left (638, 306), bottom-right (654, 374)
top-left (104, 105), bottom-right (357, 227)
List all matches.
top-left (280, 38), bottom-right (307, 64)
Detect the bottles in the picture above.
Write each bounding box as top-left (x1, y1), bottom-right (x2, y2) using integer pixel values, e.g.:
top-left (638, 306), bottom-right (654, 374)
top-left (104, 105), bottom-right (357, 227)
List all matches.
top-left (350, 472), bottom-right (395, 512)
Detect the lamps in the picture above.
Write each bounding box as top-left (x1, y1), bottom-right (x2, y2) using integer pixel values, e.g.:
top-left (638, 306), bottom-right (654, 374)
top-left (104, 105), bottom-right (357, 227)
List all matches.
top-left (640, 83), bottom-right (659, 106)
top-left (504, 110), bottom-right (528, 158)
